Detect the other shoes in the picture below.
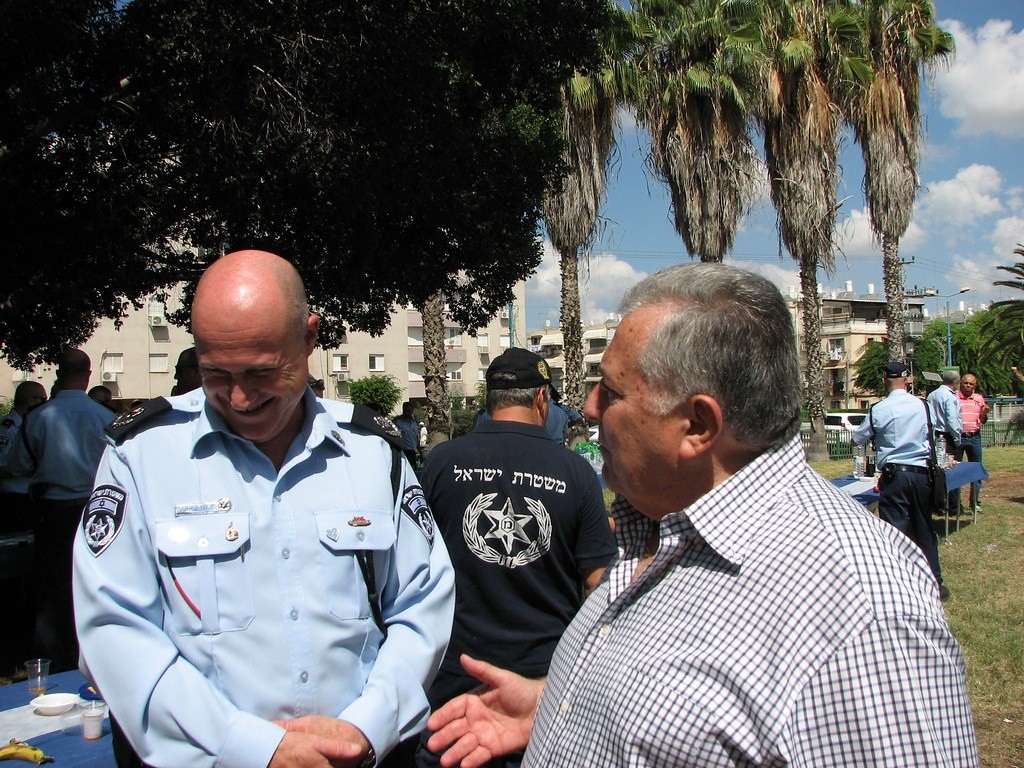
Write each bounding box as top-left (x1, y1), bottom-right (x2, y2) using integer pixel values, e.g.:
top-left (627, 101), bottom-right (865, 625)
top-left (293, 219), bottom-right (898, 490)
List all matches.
top-left (939, 584), bottom-right (949, 602)
top-left (935, 508), bottom-right (946, 515)
top-left (971, 504), bottom-right (984, 513)
top-left (951, 510), bottom-right (973, 516)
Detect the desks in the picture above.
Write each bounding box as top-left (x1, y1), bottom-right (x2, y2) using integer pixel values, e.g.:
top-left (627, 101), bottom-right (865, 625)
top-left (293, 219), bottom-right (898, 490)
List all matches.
top-left (0, 669), bottom-right (117, 768)
top-left (829, 463), bottom-right (989, 546)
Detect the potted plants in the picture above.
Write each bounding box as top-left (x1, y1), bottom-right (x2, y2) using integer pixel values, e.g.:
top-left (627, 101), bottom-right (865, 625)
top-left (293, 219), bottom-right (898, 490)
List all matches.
top-left (449, 340), bottom-right (454, 349)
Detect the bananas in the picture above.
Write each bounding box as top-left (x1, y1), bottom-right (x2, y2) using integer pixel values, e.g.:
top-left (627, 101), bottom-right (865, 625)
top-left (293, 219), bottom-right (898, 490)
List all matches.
top-left (0, 744), bottom-right (45, 764)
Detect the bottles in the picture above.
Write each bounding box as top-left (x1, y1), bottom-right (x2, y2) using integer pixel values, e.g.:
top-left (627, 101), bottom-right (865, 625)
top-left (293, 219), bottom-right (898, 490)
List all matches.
top-left (936, 434), bottom-right (946, 467)
top-left (865, 441), bottom-right (875, 477)
top-left (852, 444), bottom-right (864, 480)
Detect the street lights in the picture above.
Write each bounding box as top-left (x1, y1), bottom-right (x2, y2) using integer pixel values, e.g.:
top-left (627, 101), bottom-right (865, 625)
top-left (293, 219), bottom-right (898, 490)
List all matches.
top-left (924, 287), bottom-right (971, 367)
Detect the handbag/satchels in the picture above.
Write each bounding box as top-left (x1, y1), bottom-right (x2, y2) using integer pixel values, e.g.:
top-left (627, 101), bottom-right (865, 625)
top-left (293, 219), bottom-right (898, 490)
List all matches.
top-left (930, 466), bottom-right (945, 512)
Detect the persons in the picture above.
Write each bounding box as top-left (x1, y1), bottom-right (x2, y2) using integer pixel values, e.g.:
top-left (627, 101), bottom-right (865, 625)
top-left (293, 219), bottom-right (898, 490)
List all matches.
top-left (1011, 367), bottom-right (1024, 382)
top-left (927, 370), bottom-right (971, 516)
top-left (394, 402), bottom-right (424, 467)
top-left (89, 386), bottom-right (111, 402)
top-left (171, 348), bottom-right (203, 397)
top-left (422, 347), bottom-right (615, 768)
top-left (473, 401), bottom-right (582, 445)
top-left (956, 374), bottom-right (990, 513)
top-left (71, 249), bottom-right (454, 767)
top-left (5, 349), bottom-right (117, 671)
top-left (426, 262), bottom-right (979, 767)
top-left (419, 422), bottom-right (428, 447)
top-left (0, 381), bottom-right (48, 537)
top-left (850, 362), bottom-right (949, 600)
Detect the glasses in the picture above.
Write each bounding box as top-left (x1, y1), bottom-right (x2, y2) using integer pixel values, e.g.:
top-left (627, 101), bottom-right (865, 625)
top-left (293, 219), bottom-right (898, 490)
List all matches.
top-left (26, 396), bottom-right (47, 401)
top-left (95, 400), bottom-right (107, 405)
top-left (175, 365), bottom-right (198, 373)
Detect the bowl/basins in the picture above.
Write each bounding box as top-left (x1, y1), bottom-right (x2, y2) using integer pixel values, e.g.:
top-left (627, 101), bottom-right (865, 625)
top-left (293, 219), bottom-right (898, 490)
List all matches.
top-left (29, 692), bottom-right (79, 716)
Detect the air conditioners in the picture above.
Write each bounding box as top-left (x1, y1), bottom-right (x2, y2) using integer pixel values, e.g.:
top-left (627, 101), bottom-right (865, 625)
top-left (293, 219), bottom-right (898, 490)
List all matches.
top-left (102, 371), bottom-right (117, 382)
top-left (336, 372), bottom-right (346, 383)
top-left (500, 311), bottom-right (509, 319)
top-left (152, 316), bottom-right (168, 327)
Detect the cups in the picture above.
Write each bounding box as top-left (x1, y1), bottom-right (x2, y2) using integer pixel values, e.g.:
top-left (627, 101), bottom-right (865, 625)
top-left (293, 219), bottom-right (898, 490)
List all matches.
top-left (25, 659), bottom-right (51, 699)
top-left (78, 700), bottom-right (106, 740)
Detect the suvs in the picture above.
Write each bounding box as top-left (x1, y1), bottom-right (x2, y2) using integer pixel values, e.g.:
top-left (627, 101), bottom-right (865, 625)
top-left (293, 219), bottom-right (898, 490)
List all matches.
top-left (825, 412), bottom-right (869, 447)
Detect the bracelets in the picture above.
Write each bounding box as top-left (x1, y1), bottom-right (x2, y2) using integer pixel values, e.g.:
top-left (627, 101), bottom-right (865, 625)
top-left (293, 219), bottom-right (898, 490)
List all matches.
top-left (361, 749), bottom-right (375, 767)
top-left (1015, 371), bottom-right (1022, 378)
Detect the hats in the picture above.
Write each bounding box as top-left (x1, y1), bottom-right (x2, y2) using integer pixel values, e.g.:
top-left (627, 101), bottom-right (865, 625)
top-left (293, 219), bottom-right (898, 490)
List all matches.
top-left (882, 362), bottom-right (907, 378)
top-left (486, 347), bottom-right (562, 406)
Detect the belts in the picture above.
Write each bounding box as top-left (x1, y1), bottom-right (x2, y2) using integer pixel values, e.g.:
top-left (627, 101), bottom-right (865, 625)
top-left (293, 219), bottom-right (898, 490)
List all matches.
top-left (934, 431), bottom-right (965, 437)
top-left (895, 465), bottom-right (929, 475)
top-left (965, 432), bottom-right (978, 437)
